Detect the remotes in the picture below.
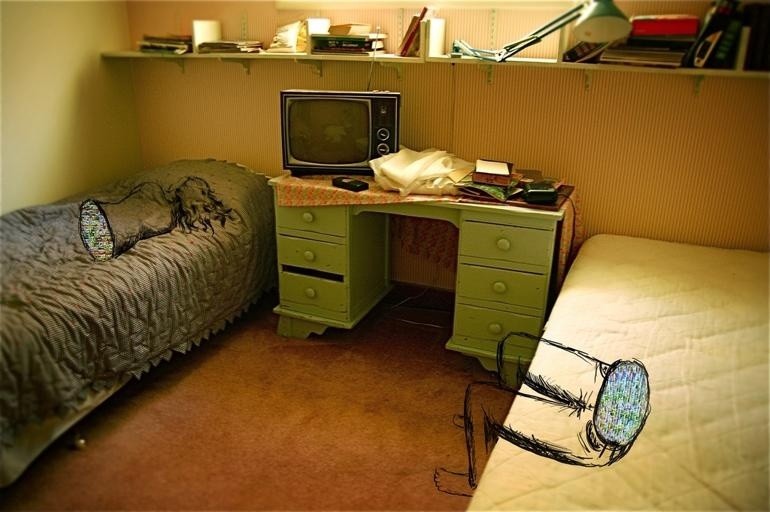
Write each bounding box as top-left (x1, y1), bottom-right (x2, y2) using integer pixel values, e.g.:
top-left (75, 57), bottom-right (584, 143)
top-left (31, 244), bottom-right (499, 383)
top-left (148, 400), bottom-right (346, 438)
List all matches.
top-left (333, 176), bottom-right (369, 192)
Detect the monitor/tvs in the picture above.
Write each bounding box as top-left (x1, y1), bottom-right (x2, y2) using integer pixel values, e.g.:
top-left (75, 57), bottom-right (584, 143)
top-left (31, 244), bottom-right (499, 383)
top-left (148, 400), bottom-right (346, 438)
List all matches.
top-left (279, 89), bottom-right (401, 177)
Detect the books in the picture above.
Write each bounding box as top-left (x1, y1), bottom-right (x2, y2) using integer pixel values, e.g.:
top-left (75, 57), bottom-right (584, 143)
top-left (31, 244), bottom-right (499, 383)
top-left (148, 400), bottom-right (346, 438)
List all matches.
top-left (198, 40), bottom-right (262, 53)
top-left (137, 33), bottom-right (191, 55)
top-left (396, 6), bottom-right (434, 56)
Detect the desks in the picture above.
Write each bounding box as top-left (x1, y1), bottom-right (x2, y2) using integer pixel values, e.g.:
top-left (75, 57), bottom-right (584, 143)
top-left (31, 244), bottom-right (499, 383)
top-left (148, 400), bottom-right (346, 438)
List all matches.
top-left (269, 172), bottom-right (577, 386)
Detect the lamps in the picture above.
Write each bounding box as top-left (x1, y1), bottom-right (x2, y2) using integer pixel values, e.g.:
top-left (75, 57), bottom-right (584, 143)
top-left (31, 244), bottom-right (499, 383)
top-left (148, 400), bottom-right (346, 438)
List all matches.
top-left (450, 0), bottom-right (632, 64)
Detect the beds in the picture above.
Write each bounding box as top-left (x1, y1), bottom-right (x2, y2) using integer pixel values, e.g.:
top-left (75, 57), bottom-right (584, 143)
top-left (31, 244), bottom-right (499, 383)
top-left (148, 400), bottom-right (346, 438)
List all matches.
top-left (465, 235), bottom-right (770, 511)
top-left (0, 160), bottom-right (276, 487)
